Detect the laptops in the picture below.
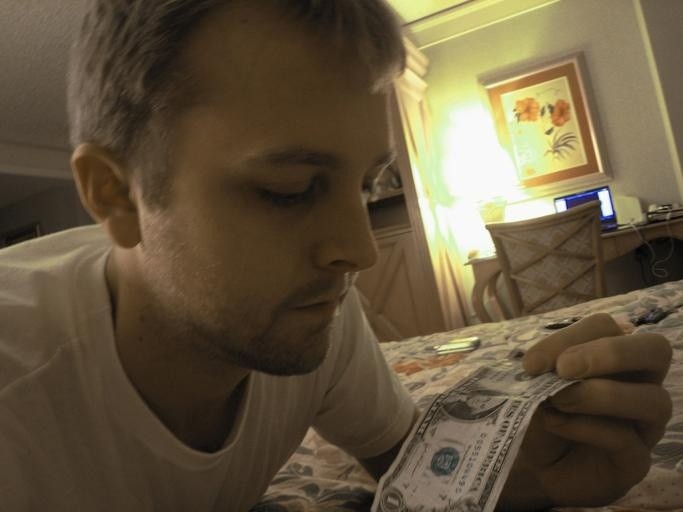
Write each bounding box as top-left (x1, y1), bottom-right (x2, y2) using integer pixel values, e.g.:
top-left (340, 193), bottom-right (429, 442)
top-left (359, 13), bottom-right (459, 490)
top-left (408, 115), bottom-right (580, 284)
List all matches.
top-left (553, 186), bottom-right (619, 231)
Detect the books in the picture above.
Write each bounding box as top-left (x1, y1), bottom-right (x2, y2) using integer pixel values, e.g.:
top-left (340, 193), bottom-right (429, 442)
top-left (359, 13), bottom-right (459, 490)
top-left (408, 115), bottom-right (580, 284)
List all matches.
top-left (643, 206), bottom-right (683, 222)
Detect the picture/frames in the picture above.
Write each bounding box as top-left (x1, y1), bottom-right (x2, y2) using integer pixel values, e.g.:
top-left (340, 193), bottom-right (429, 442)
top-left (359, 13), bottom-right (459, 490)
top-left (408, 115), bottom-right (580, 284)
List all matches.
top-left (1, 221), bottom-right (43, 247)
top-left (475, 48), bottom-right (615, 205)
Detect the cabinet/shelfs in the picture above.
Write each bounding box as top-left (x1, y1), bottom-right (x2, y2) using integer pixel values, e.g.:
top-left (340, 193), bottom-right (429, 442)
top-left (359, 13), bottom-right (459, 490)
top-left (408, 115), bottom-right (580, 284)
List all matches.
top-left (356, 38), bottom-right (472, 342)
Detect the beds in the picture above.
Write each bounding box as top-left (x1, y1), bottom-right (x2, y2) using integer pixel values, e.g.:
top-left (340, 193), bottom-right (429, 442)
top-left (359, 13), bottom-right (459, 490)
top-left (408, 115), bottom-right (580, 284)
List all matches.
top-left (258, 279), bottom-right (683, 512)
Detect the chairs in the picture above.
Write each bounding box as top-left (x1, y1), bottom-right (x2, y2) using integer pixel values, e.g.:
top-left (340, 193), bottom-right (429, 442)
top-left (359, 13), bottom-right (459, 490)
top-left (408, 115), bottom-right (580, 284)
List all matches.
top-left (485, 200), bottom-right (607, 318)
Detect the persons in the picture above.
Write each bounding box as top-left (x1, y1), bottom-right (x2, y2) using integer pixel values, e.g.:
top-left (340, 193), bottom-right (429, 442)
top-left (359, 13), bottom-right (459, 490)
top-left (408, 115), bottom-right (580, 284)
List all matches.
top-left (446, 385), bottom-right (510, 419)
top-left (0, 0), bottom-right (676, 511)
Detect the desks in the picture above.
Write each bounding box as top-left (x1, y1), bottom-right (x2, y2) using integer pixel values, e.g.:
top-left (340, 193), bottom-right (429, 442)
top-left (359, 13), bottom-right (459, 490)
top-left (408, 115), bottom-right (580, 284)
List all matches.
top-left (464, 216), bottom-right (683, 323)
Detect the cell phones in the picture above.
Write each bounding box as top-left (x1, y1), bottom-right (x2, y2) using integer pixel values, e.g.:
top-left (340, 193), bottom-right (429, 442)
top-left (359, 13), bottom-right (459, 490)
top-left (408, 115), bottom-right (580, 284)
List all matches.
top-left (425, 336), bottom-right (480, 355)
